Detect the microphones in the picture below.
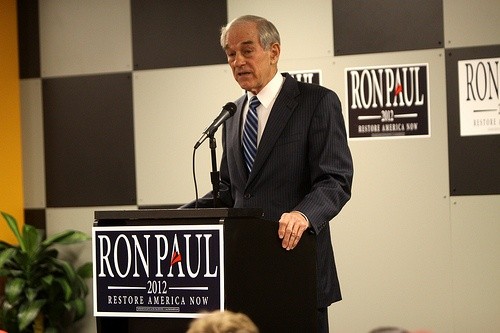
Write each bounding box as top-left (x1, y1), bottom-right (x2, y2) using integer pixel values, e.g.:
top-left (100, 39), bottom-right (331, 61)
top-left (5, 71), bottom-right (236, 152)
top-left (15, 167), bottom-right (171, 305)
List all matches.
top-left (194, 102), bottom-right (237, 149)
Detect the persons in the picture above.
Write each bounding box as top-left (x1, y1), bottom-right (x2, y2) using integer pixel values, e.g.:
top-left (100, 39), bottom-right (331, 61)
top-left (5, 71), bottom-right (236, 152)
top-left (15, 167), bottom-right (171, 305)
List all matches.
top-left (177, 15), bottom-right (353, 333)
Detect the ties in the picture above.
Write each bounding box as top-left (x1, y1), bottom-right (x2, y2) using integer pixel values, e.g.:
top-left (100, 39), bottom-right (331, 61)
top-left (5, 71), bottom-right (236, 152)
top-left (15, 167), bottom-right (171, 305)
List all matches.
top-left (240, 96), bottom-right (263, 173)
